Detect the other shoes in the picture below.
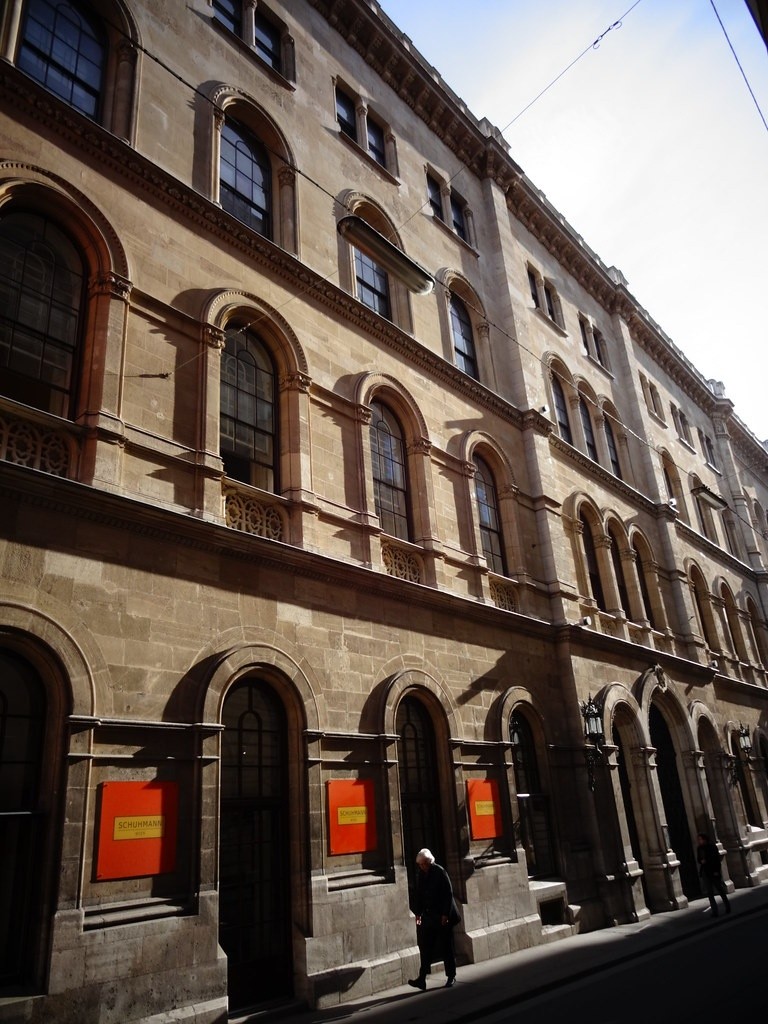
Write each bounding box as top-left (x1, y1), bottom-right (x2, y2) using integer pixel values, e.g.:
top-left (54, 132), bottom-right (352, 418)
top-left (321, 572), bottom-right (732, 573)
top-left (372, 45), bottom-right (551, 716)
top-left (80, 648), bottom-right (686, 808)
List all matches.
top-left (408, 978), bottom-right (427, 990)
top-left (726, 904), bottom-right (732, 914)
top-left (710, 909), bottom-right (719, 917)
top-left (445, 978), bottom-right (456, 986)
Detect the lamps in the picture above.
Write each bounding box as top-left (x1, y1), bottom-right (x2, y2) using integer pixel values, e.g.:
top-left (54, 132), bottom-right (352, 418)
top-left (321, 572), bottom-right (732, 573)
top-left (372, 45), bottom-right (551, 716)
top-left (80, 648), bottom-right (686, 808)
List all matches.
top-left (581, 691), bottom-right (605, 793)
top-left (729, 722), bottom-right (754, 787)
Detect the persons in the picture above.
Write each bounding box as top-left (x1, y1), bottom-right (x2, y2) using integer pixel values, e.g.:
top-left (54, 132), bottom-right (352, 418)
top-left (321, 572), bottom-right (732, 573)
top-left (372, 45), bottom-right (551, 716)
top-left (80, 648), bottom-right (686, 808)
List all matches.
top-left (408, 848), bottom-right (461, 990)
